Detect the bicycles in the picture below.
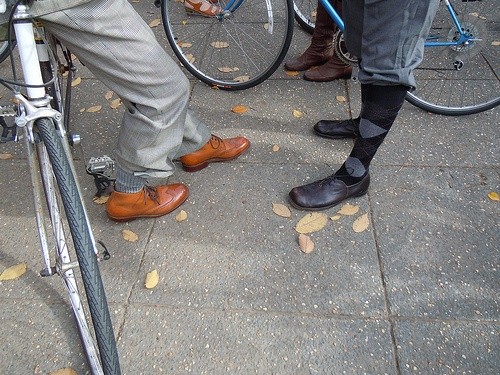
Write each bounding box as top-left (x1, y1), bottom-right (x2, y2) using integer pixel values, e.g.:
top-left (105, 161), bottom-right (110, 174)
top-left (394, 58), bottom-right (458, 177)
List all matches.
top-left (154, 0), bottom-right (500, 116)
top-left (0, 1), bottom-right (122, 375)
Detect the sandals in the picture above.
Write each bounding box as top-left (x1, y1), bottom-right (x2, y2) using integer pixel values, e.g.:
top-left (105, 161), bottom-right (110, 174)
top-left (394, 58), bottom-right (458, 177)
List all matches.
top-left (184, 0), bottom-right (224, 18)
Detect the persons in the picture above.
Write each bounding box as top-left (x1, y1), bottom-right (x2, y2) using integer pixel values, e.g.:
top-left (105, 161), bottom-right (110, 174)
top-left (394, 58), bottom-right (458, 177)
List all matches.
top-left (283, 0), bottom-right (442, 210)
top-left (184, 0), bottom-right (225, 18)
top-left (28, 0), bottom-right (250, 222)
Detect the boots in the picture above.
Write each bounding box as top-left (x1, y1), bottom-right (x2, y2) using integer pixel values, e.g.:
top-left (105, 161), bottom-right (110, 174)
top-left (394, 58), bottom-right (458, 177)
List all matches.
top-left (285, 0), bottom-right (353, 81)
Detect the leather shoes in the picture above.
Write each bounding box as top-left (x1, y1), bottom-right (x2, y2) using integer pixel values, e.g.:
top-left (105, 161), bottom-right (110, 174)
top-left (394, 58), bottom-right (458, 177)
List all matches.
top-left (106, 183), bottom-right (189, 222)
top-left (288, 170), bottom-right (371, 210)
top-left (180, 134), bottom-right (250, 172)
top-left (313, 116), bottom-right (362, 139)
